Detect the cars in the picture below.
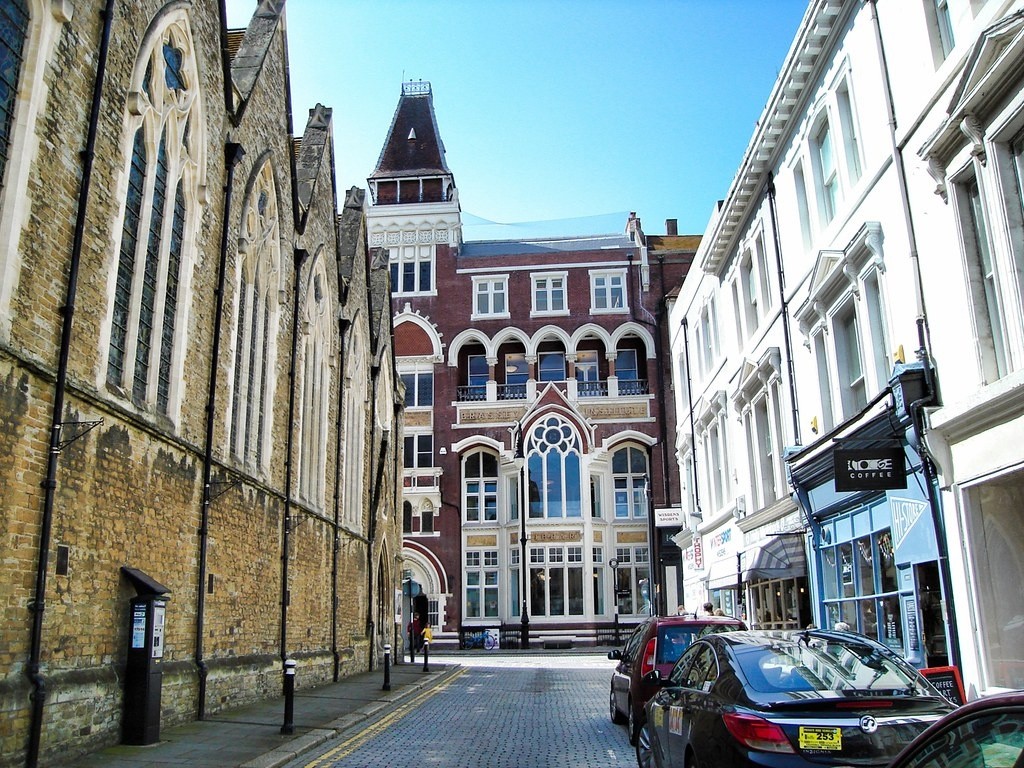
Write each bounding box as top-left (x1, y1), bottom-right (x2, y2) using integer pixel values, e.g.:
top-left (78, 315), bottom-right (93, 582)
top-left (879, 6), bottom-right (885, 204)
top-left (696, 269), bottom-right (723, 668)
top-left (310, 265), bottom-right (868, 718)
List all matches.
top-left (890, 689), bottom-right (1024, 768)
top-left (634, 629), bottom-right (982, 768)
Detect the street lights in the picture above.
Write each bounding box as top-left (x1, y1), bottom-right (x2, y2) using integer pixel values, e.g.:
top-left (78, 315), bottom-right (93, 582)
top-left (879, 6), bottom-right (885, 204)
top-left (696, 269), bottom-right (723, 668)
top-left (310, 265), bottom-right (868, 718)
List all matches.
top-left (513, 427), bottom-right (530, 649)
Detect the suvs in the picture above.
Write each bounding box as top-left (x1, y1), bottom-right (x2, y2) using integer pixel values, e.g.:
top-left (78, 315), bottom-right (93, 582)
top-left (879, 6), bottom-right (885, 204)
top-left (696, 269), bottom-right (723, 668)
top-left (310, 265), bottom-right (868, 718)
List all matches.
top-left (607, 614), bottom-right (749, 746)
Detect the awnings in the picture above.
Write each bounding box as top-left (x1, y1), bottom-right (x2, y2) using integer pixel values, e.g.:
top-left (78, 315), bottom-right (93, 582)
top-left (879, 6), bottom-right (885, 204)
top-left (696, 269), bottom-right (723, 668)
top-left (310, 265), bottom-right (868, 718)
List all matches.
top-left (707, 530), bottom-right (807, 592)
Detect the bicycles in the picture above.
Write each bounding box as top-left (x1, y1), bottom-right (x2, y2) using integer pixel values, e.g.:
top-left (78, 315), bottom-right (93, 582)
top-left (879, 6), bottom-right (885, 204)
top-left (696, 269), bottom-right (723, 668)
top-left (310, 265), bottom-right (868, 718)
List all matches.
top-left (460, 629), bottom-right (495, 651)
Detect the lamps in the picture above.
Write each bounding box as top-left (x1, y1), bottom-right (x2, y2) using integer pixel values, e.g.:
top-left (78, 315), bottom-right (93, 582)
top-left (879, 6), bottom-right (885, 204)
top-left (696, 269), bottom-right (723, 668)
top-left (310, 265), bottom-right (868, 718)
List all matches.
top-left (507, 356), bottom-right (519, 372)
top-left (577, 355), bottom-right (591, 371)
top-left (536, 569), bottom-right (551, 580)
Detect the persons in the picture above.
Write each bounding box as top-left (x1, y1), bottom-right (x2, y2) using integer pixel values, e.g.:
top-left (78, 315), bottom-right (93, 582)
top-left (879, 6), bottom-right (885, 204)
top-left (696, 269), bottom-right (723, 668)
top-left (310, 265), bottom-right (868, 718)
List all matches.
top-left (856, 599), bottom-right (878, 641)
top-left (807, 622), bottom-right (852, 655)
top-left (407, 612), bottom-right (432, 654)
top-left (671, 602), bottom-right (797, 646)
top-left (639, 578), bottom-right (650, 615)
top-left (884, 598), bottom-right (902, 639)
